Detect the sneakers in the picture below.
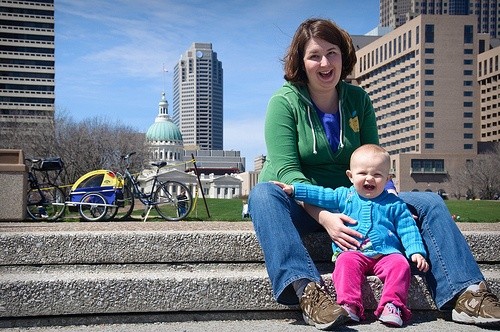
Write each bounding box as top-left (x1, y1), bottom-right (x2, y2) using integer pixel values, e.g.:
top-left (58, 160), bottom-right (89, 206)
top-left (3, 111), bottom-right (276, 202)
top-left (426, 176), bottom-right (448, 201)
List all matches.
top-left (300, 282), bottom-right (349, 330)
top-left (378, 303), bottom-right (403, 326)
top-left (341, 303), bottom-right (359, 321)
top-left (452, 280), bottom-right (500, 325)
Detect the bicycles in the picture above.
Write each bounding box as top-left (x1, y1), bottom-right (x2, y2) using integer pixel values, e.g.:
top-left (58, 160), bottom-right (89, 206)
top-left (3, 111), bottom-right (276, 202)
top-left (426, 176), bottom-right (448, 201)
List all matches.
top-left (25, 158), bottom-right (66, 222)
top-left (95, 152), bottom-right (194, 222)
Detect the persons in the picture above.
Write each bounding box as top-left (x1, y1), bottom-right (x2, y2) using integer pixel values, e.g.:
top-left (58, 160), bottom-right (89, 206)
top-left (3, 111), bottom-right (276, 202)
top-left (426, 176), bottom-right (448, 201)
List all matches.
top-left (247, 17), bottom-right (500, 330)
top-left (268, 143), bottom-right (430, 328)
